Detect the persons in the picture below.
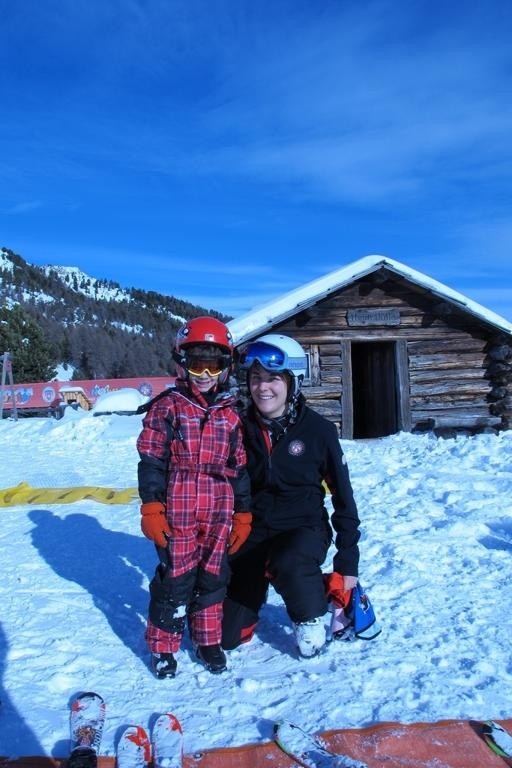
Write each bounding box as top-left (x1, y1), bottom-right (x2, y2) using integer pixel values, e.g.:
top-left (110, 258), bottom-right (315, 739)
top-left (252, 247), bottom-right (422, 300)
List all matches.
top-left (137, 317), bottom-right (253, 679)
top-left (221, 334), bottom-right (359, 659)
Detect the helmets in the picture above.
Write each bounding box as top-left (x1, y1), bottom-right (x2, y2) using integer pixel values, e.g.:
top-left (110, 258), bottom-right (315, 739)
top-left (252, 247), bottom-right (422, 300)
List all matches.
top-left (238, 333), bottom-right (308, 402)
top-left (175, 316), bottom-right (235, 385)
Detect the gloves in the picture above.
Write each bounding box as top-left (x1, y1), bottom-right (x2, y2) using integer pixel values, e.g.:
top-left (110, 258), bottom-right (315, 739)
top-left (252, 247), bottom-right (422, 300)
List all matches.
top-left (140, 502), bottom-right (172, 549)
top-left (227, 512), bottom-right (253, 555)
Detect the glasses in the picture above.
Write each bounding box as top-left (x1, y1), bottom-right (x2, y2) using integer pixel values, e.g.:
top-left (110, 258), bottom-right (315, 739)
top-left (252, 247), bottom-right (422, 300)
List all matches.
top-left (171, 347), bottom-right (234, 377)
top-left (238, 341), bottom-right (307, 372)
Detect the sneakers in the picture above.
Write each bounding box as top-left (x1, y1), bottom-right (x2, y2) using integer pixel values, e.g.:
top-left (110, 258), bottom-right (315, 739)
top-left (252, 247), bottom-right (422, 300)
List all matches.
top-left (151, 651), bottom-right (177, 679)
top-left (192, 643), bottom-right (227, 674)
top-left (294, 616), bottom-right (327, 659)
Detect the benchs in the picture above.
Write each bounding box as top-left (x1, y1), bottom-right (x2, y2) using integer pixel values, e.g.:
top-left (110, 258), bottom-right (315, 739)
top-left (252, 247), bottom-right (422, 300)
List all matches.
top-left (428, 413), bottom-right (502, 438)
